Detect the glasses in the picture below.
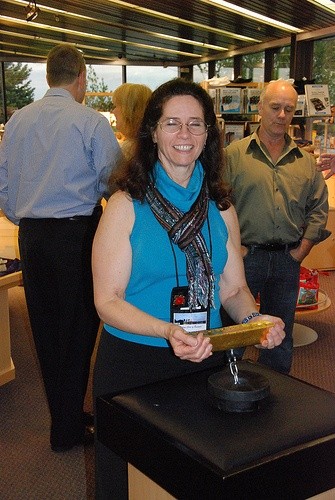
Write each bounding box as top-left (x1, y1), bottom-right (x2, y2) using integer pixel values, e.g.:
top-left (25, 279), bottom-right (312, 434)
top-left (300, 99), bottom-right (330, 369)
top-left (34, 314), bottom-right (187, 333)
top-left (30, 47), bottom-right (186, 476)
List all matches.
top-left (155, 119), bottom-right (211, 136)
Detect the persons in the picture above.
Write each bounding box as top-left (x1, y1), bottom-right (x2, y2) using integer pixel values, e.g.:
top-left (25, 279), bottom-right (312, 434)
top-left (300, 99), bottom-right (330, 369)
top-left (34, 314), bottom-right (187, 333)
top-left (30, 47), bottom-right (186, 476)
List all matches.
top-left (110, 83), bottom-right (154, 160)
top-left (0, 45), bottom-right (132, 453)
top-left (313, 153), bottom-right (335, 180)
top-left (80, 77), bottom-right (287, 498)
top-left (218, 79), bottom-right (332, 376)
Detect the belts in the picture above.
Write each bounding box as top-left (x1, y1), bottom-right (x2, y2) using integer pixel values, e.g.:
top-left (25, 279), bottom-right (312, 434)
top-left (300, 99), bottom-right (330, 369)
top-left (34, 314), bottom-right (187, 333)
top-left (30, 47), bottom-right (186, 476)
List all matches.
top-left (241, 241), bottom-right (301, 252)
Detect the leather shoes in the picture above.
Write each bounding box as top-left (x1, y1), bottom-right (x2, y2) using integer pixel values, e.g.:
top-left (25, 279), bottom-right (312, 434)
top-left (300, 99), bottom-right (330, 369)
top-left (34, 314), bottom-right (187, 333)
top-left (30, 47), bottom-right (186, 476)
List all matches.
top-left (50, 412), bottom-right (97, 451)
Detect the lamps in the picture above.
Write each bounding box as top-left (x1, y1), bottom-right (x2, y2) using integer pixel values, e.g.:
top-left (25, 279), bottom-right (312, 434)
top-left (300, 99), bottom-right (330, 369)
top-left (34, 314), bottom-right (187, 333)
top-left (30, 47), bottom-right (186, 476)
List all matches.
top-left (24, 0), bottom-right (40, 22)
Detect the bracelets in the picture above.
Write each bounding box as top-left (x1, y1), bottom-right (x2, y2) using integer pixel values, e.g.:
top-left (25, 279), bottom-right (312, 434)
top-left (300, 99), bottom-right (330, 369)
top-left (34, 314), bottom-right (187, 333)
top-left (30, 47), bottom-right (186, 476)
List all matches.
top-left (240, 312), bottom-right (262, 324)
